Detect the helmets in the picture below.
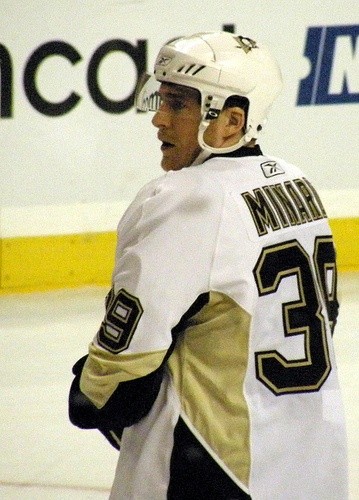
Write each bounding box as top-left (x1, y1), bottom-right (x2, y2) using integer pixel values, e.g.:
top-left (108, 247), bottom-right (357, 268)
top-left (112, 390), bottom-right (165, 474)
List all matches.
top-left (153, 31), bottom-right (283, 154)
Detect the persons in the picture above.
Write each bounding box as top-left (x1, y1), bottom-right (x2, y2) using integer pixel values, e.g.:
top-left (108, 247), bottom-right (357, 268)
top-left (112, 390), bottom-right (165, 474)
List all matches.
top-left (70, 31), bottom-right (349, 500)
